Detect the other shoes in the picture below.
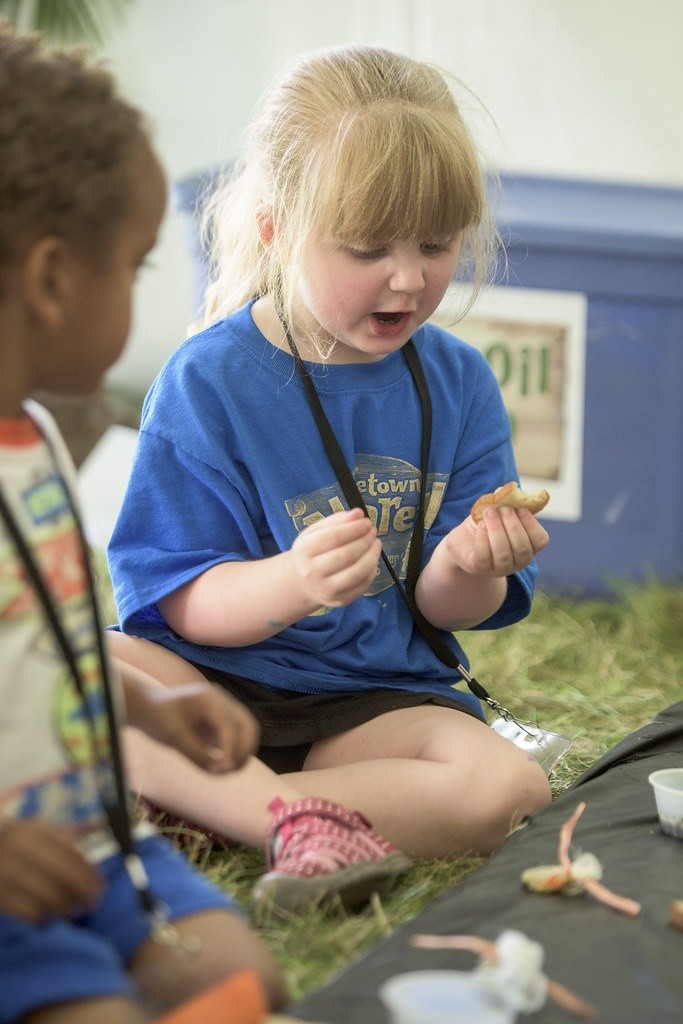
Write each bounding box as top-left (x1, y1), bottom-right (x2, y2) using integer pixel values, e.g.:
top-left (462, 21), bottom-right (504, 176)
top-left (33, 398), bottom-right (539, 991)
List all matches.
top-left (254, 798), bottom-right (414, 920)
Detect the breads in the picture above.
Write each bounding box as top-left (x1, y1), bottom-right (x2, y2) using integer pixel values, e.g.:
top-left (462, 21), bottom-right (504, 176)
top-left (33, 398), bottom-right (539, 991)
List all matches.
top-left (471, 481), bottom-right (550, 526)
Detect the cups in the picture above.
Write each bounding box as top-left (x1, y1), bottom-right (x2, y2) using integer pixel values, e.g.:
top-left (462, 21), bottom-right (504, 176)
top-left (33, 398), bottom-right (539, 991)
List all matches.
top-left (378, 969), bottom-right (518, 1024)
top-left (649, 767), bottom-right (683, 839)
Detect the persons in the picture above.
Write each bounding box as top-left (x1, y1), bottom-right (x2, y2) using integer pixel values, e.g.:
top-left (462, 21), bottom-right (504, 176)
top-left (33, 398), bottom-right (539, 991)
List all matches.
top-left (104, 47), bottom-right (553, 920)
top-left (0, 29), bottom-right (287, 1024)
